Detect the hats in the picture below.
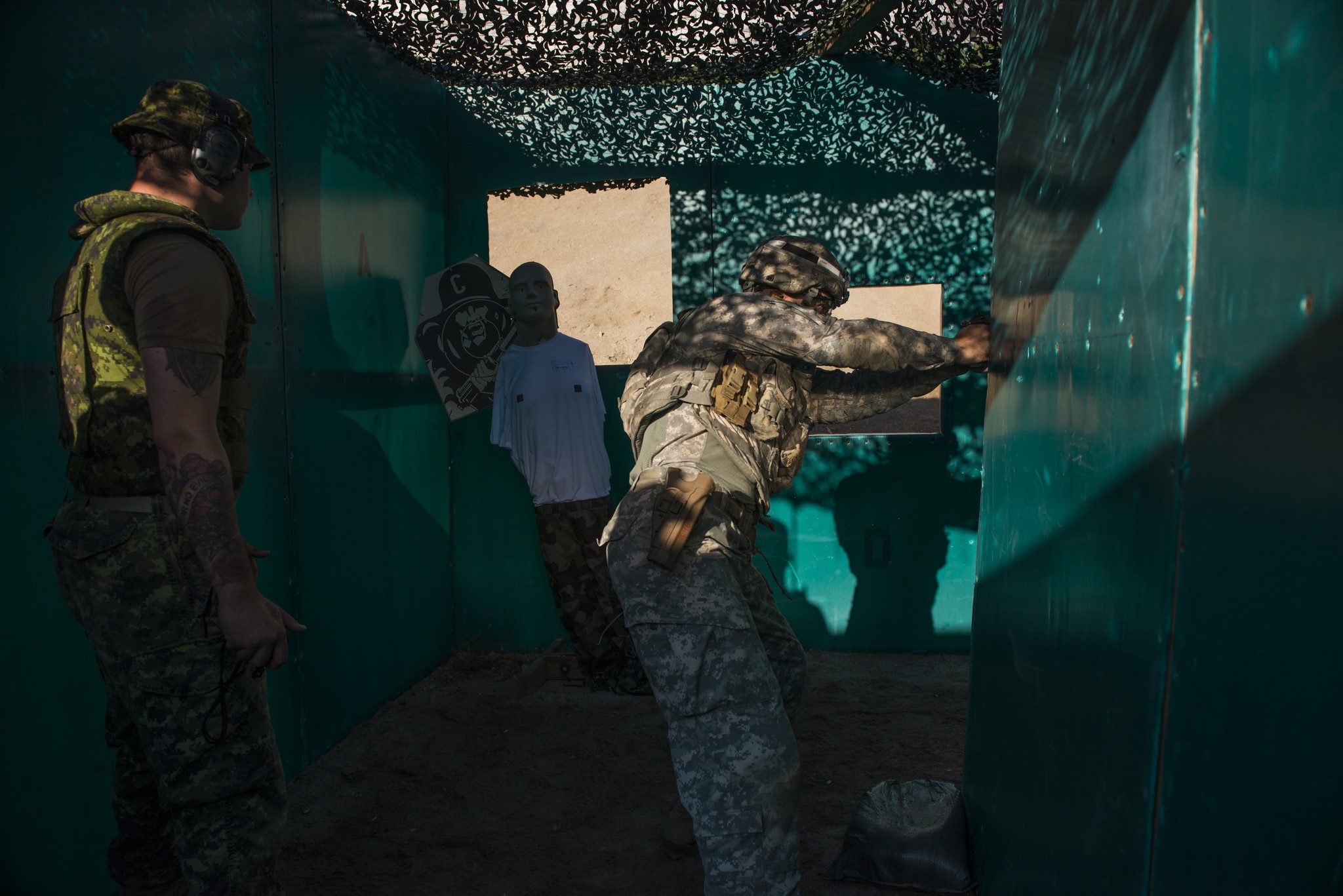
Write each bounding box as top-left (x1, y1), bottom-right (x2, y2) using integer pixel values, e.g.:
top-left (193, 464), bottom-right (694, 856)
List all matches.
top-left (113, 78), bottom-right (273, 174)
top-left (738, 235), bottom-right (850, 307)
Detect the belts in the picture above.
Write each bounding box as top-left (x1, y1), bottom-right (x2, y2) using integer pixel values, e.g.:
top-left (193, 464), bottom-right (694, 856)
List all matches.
top-left (67, 494), bottom-right (175, 514)
top-left (639, 465), bottom-right (759, 529)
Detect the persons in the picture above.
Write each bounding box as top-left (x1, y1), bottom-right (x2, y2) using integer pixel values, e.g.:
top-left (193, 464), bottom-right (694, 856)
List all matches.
top-left (592, 232), bottom-right (988, 895)
top-left (491, 261), bottom-right (645, 691)
top-left (44, 80), bottom-right (306, 896)
